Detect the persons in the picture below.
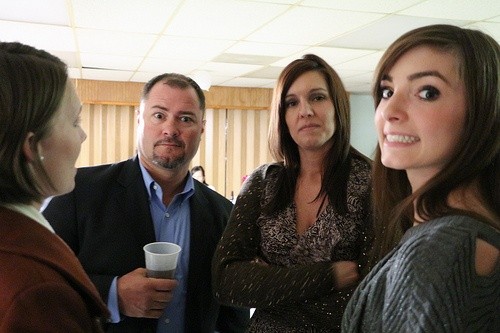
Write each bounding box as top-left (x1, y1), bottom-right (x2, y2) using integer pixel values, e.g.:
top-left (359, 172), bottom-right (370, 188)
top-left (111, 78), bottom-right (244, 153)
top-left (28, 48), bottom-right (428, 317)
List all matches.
top-left (341, 25), bottom-right (500, 333)
top-left (211, 52), bottom-right (379, 333)
top-left (191, 165), bottom-right (217, 192)
top-left (0, 41), bottom-right (112, 332)
top-left (41, 72), bottom-right (237, 333)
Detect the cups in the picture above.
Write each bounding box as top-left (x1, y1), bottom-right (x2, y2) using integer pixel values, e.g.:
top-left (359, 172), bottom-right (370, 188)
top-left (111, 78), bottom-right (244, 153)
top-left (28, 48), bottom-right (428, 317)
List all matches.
top-left (143, 242), bottom-right (182, 279)
